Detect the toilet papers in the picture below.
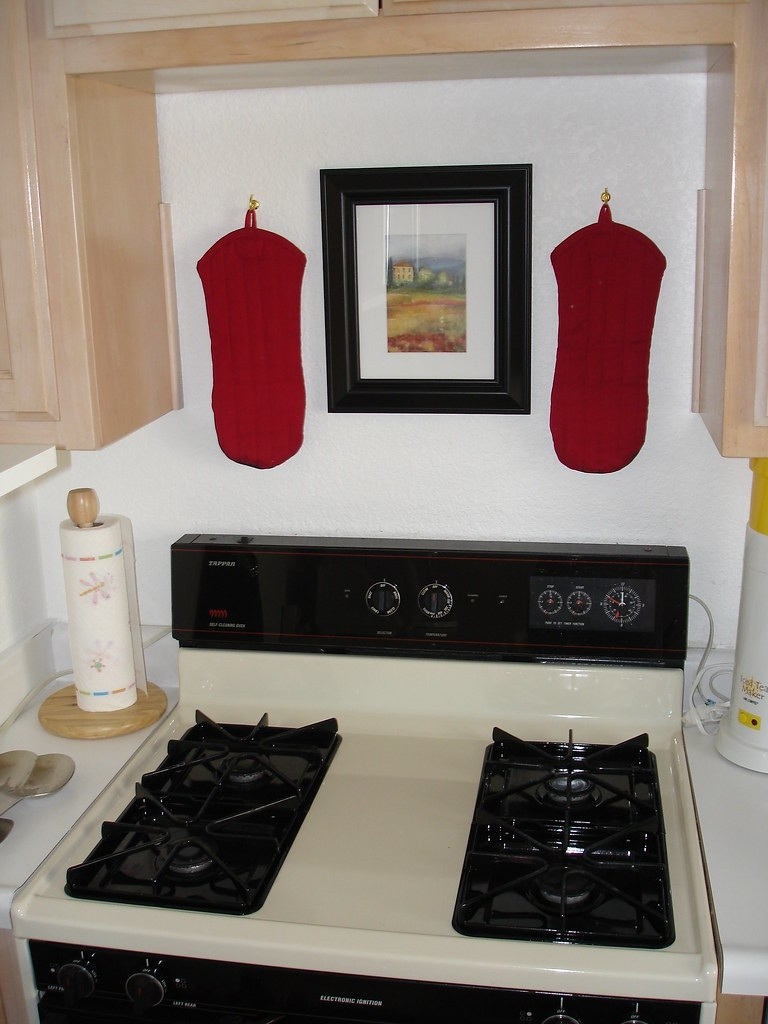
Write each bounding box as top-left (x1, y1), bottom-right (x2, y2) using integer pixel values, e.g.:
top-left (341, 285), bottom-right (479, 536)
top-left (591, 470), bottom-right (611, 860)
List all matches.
top-left (60, 515), bottom-right (150, 714)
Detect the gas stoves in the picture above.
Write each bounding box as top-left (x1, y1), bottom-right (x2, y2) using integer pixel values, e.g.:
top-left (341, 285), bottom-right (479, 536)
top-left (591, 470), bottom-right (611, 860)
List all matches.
top-left (10, 533), bottom-right (718, 1024)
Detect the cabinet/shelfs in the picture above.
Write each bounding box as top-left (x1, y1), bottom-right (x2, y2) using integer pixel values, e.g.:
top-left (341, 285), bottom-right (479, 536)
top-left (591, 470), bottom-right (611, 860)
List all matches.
top-left (0, 0), bottom-right (768, 460)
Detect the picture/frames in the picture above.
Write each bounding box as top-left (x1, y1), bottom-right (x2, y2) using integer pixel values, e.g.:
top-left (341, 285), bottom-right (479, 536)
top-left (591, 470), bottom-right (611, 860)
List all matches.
top-left (320, 164), bottom-right (533, 415)
